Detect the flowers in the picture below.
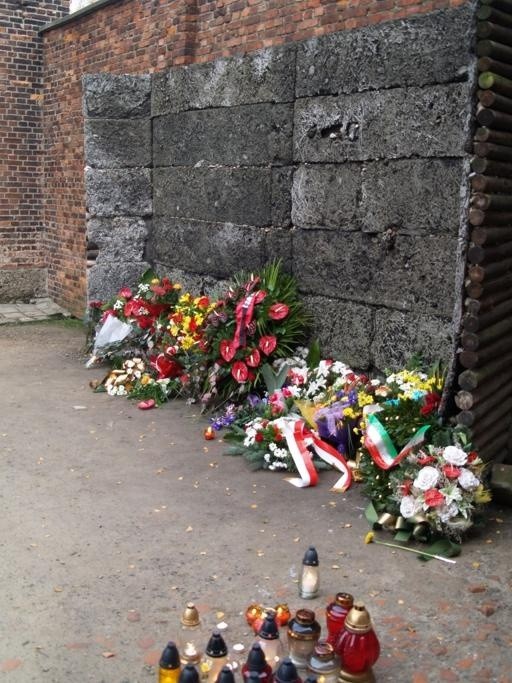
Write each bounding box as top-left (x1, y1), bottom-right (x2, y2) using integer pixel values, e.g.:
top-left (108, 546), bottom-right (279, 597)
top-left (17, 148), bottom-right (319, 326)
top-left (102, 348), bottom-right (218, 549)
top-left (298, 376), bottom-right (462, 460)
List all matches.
top-left (199, 273), bottom-right (285, 408)
top-left (83, 275), bottom-right (213, 408)
top-left (370, 432), bottom-right (494, 543)
top-left (213, 347), bottom-right (363, 474)
top-left (343, 357), bottom-right (446, 478)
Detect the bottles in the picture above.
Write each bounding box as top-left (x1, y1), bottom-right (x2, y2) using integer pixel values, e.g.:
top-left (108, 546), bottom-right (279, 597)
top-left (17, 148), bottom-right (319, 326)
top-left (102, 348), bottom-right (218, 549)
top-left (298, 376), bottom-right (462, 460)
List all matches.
top-left (157, 545), bottom-right (381, 683)
